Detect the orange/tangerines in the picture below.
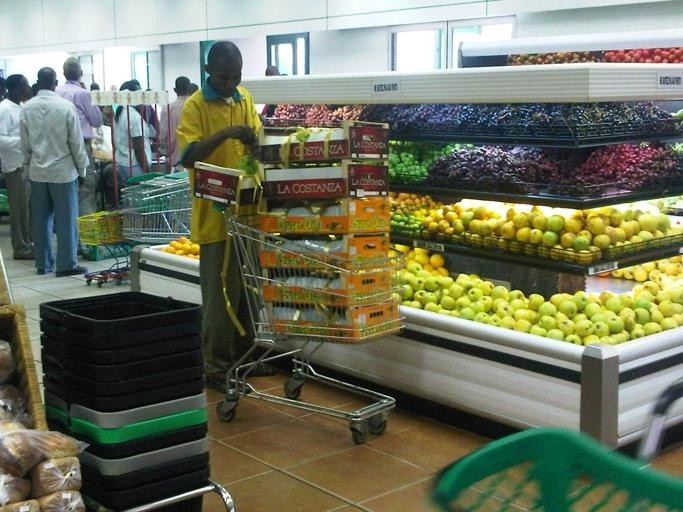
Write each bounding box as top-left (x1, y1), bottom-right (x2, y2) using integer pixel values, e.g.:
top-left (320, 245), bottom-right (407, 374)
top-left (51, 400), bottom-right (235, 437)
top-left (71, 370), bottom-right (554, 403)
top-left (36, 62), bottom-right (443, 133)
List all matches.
top-left (388, 211), bottom-right (425, 238)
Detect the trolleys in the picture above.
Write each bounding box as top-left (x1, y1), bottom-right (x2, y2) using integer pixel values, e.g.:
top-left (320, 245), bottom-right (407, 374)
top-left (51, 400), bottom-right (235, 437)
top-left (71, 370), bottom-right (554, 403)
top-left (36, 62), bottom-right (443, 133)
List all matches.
top-left (197, 194), bottom-right (406, 445)
top-left (73, 209), bottom-right (129, 287)
top-left (120, 176), bottom-right (195, 244)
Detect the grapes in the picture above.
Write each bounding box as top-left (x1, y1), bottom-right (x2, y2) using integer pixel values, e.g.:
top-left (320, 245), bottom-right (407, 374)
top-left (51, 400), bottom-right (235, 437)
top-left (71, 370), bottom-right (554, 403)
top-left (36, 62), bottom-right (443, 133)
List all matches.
top-left (359, 103), bottom-right (549, 138)
top-left (427, 143), bottom-right (680, 196)
top-left (389, 140), bottom-right (473, 185)
top-left (262, 104), bottom-right (360, 128)
top-left (547, 101), bottom-right (672, 138)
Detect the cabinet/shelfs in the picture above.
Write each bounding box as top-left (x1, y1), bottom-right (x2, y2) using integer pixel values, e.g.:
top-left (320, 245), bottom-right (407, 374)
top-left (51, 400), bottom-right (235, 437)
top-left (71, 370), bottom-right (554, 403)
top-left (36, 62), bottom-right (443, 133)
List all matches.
top-left (130, 61), bottom-right (683, 450)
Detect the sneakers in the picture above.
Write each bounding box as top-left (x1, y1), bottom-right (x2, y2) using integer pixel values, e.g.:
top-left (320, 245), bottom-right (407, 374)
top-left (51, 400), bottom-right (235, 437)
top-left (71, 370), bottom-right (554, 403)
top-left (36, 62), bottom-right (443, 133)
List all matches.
top-left (234, 361), bottom-right (278, 378)
top-left (39, 266), bottom-right (55, 274)
top-left (14, 251), bottom-right (35, 258)
top-left (206, 377), bottom-right (251, 395)
top-left (56, 266), bottom-right (87, 277)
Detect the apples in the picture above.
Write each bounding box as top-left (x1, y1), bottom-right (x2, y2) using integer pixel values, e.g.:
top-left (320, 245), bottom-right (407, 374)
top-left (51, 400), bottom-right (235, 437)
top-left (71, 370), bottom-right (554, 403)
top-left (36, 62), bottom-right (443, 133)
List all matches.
top-left (159, 235), bottom-right (201, 259)
top-left (500, 205), bottom-right (598, 265)
top-left (602, 46), bottom-right (683, 64)
top-left (570, 284), bottom-right (683, 346)
top-left (487, 285), bottom-right (586, 347)
top-left (597, 255), bottom-right (683, 287)
top-left (587, 202), bottom-right (683, 259)
top-left (427, 205), bottom-right (506, 249)
top-left (390, 261), bottom-right (495, 324)
top-left (387, 191), bottom-right (443, 215)
top-left (388, 242), bottom-right (451, 278)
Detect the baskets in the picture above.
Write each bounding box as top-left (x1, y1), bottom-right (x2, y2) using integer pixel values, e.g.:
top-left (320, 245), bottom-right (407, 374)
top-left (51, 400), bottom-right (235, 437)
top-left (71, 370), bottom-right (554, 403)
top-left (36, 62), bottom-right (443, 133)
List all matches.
top-left (431, 428), bottom-right (682, 512)
top-left (41, 291), bottom-right (210, 512)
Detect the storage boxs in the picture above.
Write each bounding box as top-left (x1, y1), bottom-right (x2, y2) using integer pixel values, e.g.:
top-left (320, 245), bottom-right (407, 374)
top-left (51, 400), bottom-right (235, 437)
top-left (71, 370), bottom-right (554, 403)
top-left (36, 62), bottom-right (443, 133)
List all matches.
top-left (257, 121), bottom-right (400, 343)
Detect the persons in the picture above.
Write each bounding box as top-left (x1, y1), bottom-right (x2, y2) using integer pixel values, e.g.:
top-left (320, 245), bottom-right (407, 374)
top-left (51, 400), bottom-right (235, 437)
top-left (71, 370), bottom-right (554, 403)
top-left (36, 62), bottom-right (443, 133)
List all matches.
top-left (161, 65), bottom-right (279, 173)
top-left (0, 57), bottom-right (160, 275)
top-left (175, 41), bottom-right (279, 395)
